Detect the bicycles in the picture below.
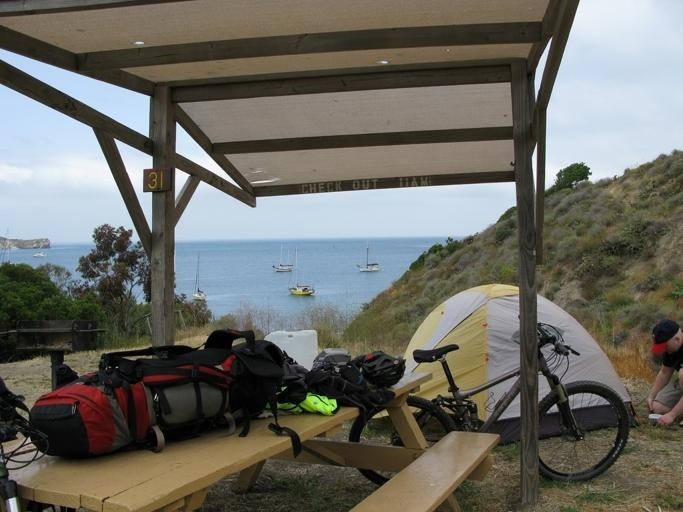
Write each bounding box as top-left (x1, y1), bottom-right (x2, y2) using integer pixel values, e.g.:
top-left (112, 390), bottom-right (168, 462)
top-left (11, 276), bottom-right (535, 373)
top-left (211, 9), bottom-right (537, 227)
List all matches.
top-left (349, 314), bottom-right (631, 483)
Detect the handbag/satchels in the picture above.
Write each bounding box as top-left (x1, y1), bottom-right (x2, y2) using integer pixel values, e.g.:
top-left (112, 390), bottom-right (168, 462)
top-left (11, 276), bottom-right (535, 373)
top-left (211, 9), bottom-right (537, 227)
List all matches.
top-left (266, 349), bottom-right (405, 421)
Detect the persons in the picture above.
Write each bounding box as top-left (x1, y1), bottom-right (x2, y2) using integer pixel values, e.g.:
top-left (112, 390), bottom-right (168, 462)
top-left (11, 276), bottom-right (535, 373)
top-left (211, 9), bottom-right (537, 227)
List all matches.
top-left (646, 320), bottom-right (683, 427)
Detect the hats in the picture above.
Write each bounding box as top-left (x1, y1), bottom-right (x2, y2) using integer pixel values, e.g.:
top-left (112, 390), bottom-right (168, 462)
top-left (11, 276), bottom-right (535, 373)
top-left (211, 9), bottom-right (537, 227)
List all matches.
top-left (650, 319), bottom-right (679, 353)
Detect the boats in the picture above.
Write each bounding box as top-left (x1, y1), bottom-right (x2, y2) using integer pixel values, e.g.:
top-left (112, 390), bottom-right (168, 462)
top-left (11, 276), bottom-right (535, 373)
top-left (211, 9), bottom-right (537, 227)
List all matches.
top-left (33, 252), bottom-right (47, 258)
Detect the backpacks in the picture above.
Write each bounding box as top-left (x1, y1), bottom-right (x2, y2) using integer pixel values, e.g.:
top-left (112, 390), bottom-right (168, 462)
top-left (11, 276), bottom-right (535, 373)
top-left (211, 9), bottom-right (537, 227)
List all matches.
top-left (27, 331), bottom-right (286, 459)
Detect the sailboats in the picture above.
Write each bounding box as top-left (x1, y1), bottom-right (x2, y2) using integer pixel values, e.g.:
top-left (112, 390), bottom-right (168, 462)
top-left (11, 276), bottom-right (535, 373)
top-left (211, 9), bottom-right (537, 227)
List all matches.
top-left (191, 256), bottom-right (208, 301)
top-left (271, 241), bottom-right (293, 272)
top-left (359, 248), bottom-right (380, 273)
top-left (288, 247), bottom-right (315, 296)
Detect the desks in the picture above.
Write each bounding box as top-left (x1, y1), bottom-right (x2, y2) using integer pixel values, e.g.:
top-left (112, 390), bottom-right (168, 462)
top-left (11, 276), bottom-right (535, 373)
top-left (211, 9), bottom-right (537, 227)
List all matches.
top-left (6, 373), bottom-right (460, 512)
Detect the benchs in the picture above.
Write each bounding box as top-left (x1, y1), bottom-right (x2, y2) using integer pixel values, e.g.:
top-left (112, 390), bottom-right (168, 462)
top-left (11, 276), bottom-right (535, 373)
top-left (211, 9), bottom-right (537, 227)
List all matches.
top-left (349, 432), bottom-right (501, 512)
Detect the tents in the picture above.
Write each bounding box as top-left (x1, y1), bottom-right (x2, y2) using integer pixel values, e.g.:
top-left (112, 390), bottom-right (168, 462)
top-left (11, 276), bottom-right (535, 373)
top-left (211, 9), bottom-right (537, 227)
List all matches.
top-left (368, 283), bottom-right (640, 446)
